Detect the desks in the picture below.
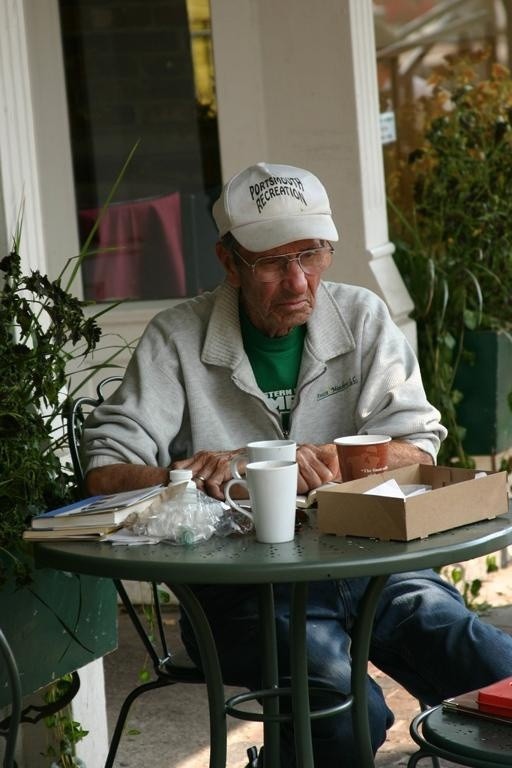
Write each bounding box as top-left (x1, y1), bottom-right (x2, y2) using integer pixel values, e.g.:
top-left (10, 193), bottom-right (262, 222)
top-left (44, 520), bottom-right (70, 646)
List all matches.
top-left (29, 515), bottom-right (512, 766)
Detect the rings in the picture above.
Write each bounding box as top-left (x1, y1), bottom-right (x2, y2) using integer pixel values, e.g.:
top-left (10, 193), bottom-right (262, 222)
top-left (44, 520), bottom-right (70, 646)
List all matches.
top-left (193, 473), bottom-right (207, 484)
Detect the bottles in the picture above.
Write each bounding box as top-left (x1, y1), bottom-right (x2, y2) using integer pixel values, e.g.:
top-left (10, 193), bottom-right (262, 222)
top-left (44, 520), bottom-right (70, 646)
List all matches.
top-left (168, 468), bottom-right (197, 493)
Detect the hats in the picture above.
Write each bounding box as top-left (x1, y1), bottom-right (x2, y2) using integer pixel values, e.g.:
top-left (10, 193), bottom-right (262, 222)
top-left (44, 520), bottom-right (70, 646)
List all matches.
top-left (210, 162), bottom-right (339, 254)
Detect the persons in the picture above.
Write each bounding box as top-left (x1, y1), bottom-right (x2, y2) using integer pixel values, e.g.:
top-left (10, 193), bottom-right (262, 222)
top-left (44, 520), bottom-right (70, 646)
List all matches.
top-left (82, 160), bottom-right (511, 767)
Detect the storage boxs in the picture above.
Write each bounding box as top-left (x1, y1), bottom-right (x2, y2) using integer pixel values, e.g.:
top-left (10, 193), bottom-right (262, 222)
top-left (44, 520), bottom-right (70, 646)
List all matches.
top-left (312, 462), bottom-right (509, 545)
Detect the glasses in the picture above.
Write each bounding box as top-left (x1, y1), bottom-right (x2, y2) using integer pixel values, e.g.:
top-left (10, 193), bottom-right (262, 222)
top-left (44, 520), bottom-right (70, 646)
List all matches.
top-left (231, 241), bottom-right (336, 284)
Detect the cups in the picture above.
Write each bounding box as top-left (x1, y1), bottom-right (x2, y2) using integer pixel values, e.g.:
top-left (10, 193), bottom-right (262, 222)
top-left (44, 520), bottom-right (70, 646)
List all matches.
top-left (332, 432), bottom-right (394, 485)
top-left (222, 459), bottom-right (300, 547)
top-left (227, 437), bottom-right (299, 490)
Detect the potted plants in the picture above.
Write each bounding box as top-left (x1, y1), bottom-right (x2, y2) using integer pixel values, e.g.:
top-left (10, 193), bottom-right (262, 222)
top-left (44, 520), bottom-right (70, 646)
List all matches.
top-left (387, 54), bottom-right (511, 457)
top-left (1, 251), bottom-right (125, 709)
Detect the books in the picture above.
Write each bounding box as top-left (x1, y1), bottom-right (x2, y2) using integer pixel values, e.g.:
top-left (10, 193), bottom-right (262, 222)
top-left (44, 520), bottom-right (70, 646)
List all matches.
top-left (477, 677), bottom-right (511, 709)
top-left (22, 521), bottom-right (125, 541)
top-left (441, 687), bottom-right (511, 727)
top-left (31, 481), bottom-right (168, 530)
top-left (225, 481), bottom-right (342, 510)
